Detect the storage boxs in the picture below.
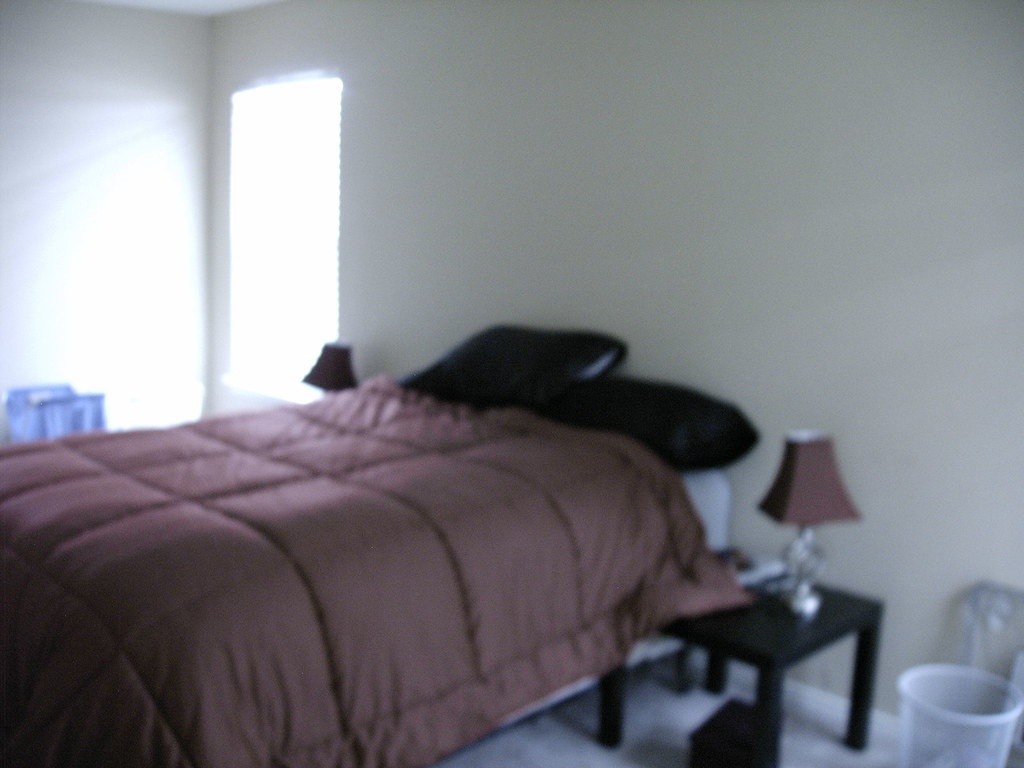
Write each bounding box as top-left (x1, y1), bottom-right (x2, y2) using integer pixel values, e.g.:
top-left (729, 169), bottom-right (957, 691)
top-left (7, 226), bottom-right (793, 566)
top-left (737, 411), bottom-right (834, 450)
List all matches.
top-left (7, 384), bottom-right (105, 443)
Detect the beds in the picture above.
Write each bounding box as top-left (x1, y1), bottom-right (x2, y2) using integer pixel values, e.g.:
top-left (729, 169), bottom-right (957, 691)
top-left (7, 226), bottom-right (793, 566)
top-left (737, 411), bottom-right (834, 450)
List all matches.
top-left (0, 325), bottom-right (760, 768)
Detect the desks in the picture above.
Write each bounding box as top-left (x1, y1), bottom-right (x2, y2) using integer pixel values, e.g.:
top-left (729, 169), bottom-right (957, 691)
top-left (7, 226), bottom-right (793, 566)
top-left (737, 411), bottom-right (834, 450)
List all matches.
top-left (597, 577), bottom-right (884, 768)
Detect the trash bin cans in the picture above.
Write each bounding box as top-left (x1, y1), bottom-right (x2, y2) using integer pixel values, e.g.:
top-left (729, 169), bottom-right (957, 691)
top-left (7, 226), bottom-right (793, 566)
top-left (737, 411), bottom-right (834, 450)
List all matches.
top-left (895, 657), bottom-right (1024, 768)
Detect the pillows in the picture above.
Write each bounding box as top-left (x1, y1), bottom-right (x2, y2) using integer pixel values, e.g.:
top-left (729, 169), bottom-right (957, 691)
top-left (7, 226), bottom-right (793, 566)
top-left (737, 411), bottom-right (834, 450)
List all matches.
top-left (398, 325), bottom-right (760, 473)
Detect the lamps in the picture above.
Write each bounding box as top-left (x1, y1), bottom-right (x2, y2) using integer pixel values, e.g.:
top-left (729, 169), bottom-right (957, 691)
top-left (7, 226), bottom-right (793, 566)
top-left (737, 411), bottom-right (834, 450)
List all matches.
top-left (757, 429), bottom-right (865, 619)
top-left (303, 343), bottom-right (358, 390)
